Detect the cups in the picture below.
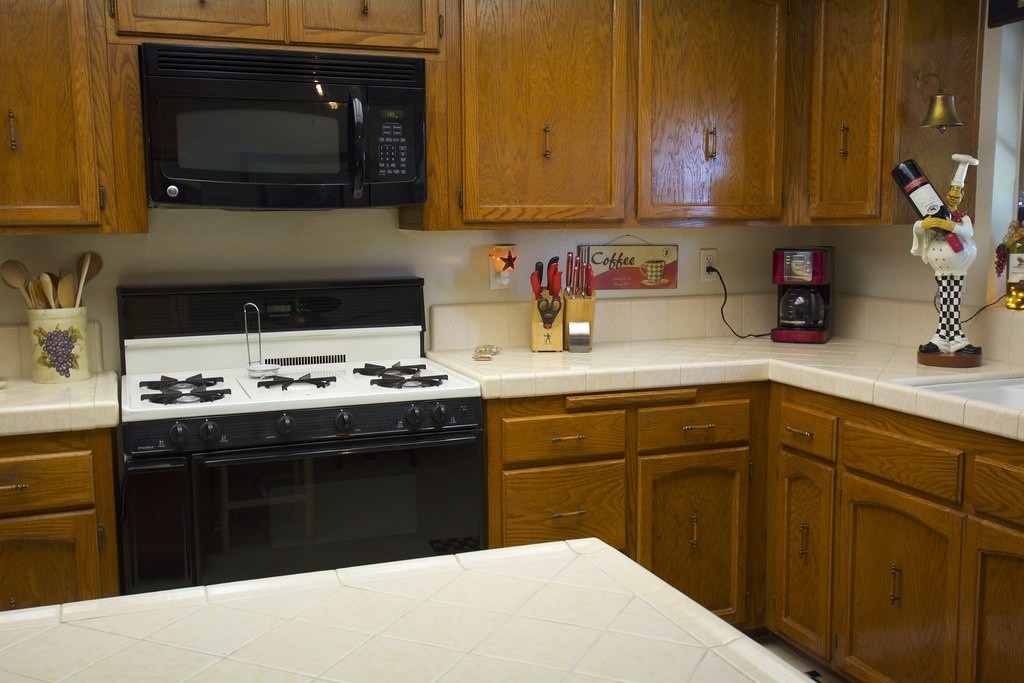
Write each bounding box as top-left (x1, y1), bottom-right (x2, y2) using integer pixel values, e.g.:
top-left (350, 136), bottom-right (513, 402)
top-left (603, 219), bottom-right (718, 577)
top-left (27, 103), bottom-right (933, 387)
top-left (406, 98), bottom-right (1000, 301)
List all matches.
top-left (640, 260), bottom-right (664, 283)
top-left (27, 307), bottom-right (90, 384)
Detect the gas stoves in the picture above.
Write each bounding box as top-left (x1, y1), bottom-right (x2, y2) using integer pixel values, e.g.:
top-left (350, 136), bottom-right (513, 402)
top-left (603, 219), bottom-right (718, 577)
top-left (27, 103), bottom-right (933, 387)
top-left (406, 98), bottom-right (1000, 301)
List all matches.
top-left (117, 276), bottom-right (480, 421)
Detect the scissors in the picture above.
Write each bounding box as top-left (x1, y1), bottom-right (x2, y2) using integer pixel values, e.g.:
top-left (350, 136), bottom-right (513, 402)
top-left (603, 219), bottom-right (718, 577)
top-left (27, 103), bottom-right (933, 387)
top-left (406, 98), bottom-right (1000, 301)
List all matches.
top-left (537, 297), bottom-right (562, 328)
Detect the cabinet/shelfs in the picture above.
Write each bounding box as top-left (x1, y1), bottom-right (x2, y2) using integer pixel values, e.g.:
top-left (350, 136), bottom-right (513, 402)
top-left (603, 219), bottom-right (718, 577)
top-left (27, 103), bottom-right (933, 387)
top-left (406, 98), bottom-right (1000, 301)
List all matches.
top-left (792, 0), bottom-right (987, 224)
top-left (427, 0), bottom-right (788, 234)
top-left (0, 1), bottom-right (145, 238)
top-left (108, 2), bottom-right (442, 50)
top-left (963, 428), bottom-right (1022, 683)
top-left (486, 385), bottom-right (776, 623)
top-left (769, 392), bottom-right (966, 683)
top-left (1, 428), bottom-right (121, 611)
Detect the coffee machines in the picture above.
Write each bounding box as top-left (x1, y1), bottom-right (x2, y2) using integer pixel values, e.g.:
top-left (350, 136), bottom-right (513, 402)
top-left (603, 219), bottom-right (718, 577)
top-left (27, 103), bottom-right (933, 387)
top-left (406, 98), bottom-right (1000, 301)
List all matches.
top-left (771, 245), bottom-right (833, 344)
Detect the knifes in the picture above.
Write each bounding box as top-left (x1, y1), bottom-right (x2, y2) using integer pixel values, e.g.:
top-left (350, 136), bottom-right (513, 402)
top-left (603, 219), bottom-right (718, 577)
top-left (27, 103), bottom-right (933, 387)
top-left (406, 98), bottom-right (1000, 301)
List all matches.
top-left (530, 252), bottom-right (593, 299)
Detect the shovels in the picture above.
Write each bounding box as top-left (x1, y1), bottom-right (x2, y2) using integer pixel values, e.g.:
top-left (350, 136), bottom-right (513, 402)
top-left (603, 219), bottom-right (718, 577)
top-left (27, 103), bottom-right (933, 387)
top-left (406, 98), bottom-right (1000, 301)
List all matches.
top-left (40, 273), bottom-right (55, 308)
top-left (58, 272), bottom-right (75, 308)
top-left (0, 260), bottom-right (34, 308)
top-left (75, 251), bottom-right (103, 306)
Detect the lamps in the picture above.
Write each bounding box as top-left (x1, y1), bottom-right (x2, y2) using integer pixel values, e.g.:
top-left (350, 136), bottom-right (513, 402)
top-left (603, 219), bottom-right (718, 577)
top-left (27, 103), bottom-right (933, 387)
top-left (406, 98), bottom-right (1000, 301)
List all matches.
top-left (493, 247), bottom-right (517, 283)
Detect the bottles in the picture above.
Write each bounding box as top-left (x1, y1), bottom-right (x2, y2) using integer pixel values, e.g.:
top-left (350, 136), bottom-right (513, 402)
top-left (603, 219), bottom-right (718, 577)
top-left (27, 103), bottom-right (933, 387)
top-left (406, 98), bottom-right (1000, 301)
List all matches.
top-left (1006, 200), bottom-right (1024, 309)
top-left (891, 159), bottom-right (964, 253)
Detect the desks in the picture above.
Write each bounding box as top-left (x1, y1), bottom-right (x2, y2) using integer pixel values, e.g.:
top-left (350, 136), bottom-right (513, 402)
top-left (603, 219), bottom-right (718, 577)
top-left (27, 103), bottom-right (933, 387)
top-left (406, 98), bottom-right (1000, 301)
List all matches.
top-left (2, 533), bottom-right (821, 682)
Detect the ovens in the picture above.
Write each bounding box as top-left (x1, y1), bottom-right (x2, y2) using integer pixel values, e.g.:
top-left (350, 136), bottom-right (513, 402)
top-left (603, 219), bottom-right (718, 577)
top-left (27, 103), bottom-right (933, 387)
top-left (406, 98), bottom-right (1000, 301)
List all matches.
top-left (121, 396), bottom-right (483, 598)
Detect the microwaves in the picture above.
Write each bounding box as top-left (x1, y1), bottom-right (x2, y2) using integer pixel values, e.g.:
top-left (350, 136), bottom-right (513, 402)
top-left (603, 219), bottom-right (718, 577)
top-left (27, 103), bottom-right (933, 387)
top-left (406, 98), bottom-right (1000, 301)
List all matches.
top-left (141, 41), bottom-right (426, 211)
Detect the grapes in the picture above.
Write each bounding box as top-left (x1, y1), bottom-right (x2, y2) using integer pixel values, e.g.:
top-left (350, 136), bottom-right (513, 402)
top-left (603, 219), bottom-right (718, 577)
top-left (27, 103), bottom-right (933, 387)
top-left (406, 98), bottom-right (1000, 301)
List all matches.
top-left (995, 244), bottom-right (1010, 278)
top-left (950, 210), bottom-right (968, 222)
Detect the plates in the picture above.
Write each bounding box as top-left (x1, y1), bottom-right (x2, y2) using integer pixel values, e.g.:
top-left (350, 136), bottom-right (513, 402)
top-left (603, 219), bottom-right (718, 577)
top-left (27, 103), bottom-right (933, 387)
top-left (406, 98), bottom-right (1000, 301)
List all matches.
top-left (641, 279), bottom-right (669, 286)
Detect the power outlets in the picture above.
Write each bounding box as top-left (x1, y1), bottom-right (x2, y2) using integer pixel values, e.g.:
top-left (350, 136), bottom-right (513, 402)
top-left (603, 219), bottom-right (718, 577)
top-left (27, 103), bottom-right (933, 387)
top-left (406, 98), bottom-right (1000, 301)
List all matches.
top-left (698, 249), bottom-right (719, 281)
top-left (488, 255), bottom-right (511, 290)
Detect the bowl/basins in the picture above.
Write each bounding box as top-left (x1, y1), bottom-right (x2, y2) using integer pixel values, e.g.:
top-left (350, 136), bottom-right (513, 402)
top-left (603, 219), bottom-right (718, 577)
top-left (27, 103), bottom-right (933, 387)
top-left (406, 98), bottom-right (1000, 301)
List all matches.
top-left (248, 364), bottom-right (280, 377)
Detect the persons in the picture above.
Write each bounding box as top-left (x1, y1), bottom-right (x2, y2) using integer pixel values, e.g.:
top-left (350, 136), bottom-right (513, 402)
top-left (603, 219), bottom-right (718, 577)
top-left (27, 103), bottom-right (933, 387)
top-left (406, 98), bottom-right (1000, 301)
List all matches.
top-left (911, 154), bottom-right (983, 355)
